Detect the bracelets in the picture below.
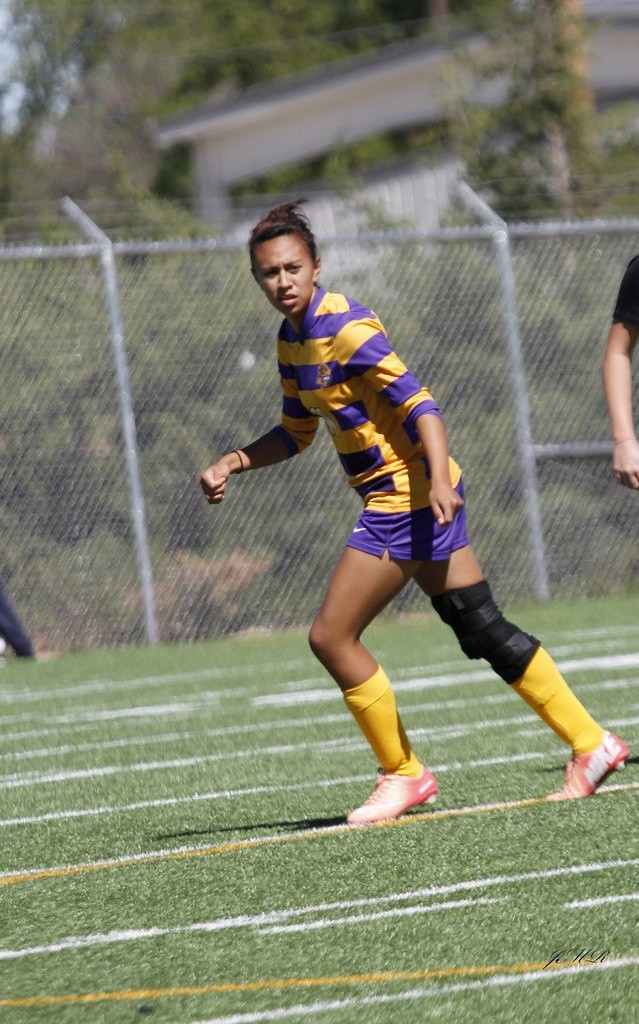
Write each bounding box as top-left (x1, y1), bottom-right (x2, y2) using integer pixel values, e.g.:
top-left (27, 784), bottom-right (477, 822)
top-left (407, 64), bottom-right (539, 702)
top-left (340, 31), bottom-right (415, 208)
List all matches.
top-left (231, 450), bottom-right (244, 474)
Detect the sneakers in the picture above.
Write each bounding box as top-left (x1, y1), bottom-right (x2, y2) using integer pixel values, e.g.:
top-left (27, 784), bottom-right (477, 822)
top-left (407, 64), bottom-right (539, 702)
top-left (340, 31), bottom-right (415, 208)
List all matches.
top-left (346, 764), bottom-right (439, 825)
top-left (546, 730), bottom-right (630, 801)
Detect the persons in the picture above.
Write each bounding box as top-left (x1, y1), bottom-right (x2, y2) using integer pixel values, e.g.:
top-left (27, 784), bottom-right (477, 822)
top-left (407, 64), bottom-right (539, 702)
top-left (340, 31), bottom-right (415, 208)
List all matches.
top-left (604, 253), bottom-right (639, 488)
top-left (0, 584), bottom-right (62, 664)
top-left (199, 198), bottom-right (628, 824)
